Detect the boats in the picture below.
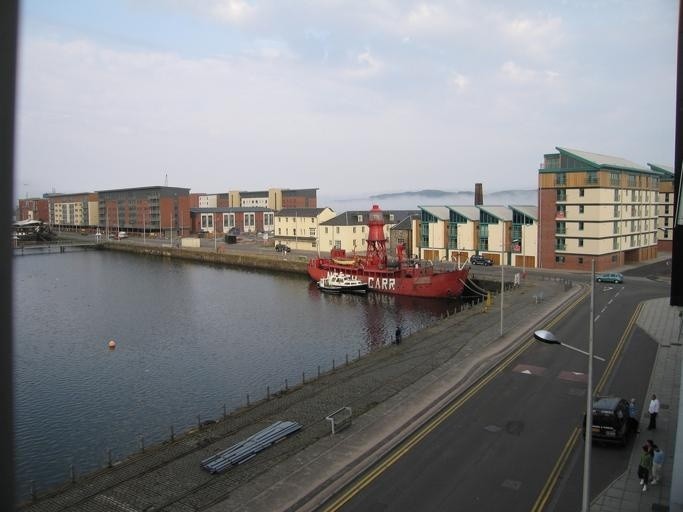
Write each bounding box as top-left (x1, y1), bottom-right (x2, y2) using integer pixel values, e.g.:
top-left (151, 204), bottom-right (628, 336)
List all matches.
top-left (308, 204), bottom-right (471, 299)
top-left (316, 270), bottom-right (367, 296)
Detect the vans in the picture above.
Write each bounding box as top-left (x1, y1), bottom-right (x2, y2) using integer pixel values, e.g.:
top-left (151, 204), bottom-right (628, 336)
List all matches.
top-left (581, 397), bottom-right (630, 445)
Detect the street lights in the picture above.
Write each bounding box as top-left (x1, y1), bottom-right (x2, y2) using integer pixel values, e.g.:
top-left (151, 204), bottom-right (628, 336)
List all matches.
top-left (532, 329), bottom-right (606, 511)
top-left (500, 239), bottom-right (519, 336)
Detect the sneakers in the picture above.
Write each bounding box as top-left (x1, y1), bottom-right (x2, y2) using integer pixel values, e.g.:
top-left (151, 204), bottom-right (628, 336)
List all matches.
top-left (639, 477), bottom-right (659, 491)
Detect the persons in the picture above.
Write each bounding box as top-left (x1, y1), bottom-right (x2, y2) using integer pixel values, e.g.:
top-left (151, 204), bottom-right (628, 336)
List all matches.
top-left (628, 398), bottom-right (640, 433)
top-left (395, 327), bottom-right (401, 345)
top-left (637, 439), bottom-right (665, 492)
top-left (646, 393), bottom-right (660, 431)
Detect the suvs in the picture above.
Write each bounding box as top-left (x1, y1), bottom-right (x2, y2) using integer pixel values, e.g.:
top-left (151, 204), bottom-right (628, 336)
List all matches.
top-left (469, 255), bottom-right (493, 266)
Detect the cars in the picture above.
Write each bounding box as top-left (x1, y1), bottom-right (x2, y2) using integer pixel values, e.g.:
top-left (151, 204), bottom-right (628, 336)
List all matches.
top-left (275, 244), bottom-right (290, 253)
top-left (594, 271), bottom-right (624, 284)
top-left (119, 232), bottom-right (128, 238)
